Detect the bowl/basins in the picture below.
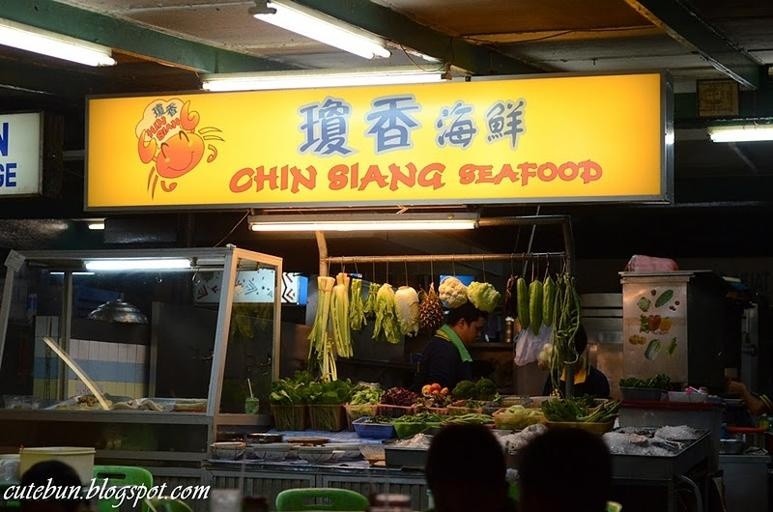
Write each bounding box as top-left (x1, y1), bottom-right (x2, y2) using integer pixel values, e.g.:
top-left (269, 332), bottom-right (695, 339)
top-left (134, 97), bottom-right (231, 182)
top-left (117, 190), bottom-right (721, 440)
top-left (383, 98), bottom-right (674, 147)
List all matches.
top-left (719, 437), bottom-right (746, 455)
top-left (209, 441), bottom-right (387, 467)
top-left (245, 433), bottom-right (284, 443)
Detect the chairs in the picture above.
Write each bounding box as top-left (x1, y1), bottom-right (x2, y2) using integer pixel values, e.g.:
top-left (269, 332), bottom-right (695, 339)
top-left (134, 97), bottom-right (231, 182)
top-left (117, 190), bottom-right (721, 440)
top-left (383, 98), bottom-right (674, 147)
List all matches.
top-left (275, 488), bottom-right (369, 512)
top-left (0, 464), bottom-right (211, 512)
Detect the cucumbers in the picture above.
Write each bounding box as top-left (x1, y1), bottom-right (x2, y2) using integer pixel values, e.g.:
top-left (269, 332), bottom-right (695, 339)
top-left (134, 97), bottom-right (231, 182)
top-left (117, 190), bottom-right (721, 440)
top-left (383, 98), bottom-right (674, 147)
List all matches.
top-left (654, 289), bottom-right (674, 308)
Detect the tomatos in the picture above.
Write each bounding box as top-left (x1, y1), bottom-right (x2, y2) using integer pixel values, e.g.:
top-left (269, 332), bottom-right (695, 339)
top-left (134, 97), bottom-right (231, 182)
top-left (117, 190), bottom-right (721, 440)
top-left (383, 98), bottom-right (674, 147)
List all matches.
top-left (421, 383), bottom-right (448, 396)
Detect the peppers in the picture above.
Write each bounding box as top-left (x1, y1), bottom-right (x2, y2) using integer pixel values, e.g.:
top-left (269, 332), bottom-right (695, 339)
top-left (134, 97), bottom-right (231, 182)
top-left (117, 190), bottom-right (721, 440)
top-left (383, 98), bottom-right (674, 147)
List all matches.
top-left (648, 314), bottom-right (673, 332)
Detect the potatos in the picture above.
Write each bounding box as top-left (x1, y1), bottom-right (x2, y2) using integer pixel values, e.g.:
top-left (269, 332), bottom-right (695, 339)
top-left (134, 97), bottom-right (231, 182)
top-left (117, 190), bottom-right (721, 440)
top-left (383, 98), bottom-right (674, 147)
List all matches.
top-left (628, 334), bottom-right (646, 345)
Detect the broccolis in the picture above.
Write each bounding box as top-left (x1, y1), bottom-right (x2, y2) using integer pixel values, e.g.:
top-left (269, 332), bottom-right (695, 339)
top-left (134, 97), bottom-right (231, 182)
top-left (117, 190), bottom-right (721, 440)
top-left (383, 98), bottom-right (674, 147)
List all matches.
top-left (638, 296), bottom-right (651, 312)
top-left (451, 377), bottom-right (497, 399)
top-left (438, 277), bottom-right (500, 313)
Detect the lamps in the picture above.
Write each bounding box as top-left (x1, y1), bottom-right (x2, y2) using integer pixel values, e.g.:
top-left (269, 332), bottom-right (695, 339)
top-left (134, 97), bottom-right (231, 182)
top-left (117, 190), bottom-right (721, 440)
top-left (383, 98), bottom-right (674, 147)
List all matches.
top-left (249, 0), bottom-right (391, 59)
top-left (247, 213), bottom-right (480, 232)
top-left (707, 125), bottom-right (773, 142)
top-left (200, 64), bottom-right (450, 92)
top-left (84, 257), bottom-right (197, 271)
top-left (86, 292), bottom-right (149, 326)
top-left (0, 18), bottom-right (117, 67)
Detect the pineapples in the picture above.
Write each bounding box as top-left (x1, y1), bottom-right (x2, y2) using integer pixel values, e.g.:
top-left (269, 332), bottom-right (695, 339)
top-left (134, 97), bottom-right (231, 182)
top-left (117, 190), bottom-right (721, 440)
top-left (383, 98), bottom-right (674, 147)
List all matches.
top-left (415, 284), bottom-right (446, 331)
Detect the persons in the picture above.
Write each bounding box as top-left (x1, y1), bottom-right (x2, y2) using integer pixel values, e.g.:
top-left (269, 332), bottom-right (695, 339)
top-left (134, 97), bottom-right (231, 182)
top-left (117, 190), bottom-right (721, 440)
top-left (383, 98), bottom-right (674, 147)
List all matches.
top-left (424, 422), bottom-right (515, 510)
top-left (726, 376), bottom-right (773, 417)
top-left (517, 425), bottom-right (612, 512)
top-left (20, 461), bottom-right (99, 511)
top-left (413, 304), bottom-right (487, 407)
top-left (544, 323), bottom-right (611, 398)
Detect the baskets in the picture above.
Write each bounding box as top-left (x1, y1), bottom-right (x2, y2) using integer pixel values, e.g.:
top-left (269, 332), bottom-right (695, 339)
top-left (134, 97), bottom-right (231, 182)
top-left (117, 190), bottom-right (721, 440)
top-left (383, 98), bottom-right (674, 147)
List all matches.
top-left (271, 393), bottom-right (616, 437)
top-left (620, 386), bottom-right (660, 400)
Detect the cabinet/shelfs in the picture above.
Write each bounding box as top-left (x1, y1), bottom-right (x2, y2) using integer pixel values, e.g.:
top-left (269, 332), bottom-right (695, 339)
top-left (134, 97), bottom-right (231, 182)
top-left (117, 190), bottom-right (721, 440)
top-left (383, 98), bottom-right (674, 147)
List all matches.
top-left (0, 247), bottom-right (283, 461)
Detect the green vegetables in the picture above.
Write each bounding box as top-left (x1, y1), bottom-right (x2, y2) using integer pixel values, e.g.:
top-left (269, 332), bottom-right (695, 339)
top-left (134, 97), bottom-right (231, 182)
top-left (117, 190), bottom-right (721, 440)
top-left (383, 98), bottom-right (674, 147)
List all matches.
top-left (306, 271), bottom-right (376, 362)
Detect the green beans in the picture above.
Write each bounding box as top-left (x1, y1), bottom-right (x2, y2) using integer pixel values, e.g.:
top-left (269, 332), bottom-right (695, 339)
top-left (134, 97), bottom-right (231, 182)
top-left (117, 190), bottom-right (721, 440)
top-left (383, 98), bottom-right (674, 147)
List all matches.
top-left (550, 267), bottom-right (588, 388)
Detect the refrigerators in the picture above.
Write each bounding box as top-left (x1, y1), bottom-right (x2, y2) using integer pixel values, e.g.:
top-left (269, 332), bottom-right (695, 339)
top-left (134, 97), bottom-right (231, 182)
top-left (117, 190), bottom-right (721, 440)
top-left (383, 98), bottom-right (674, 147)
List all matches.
top-left (617, 269), bottom-right (721, 392)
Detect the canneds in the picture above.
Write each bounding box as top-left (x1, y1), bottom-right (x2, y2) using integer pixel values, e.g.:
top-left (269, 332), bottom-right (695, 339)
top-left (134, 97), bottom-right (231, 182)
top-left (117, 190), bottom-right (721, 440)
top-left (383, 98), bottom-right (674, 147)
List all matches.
top-left (244, 397), bottom-right (260, 414)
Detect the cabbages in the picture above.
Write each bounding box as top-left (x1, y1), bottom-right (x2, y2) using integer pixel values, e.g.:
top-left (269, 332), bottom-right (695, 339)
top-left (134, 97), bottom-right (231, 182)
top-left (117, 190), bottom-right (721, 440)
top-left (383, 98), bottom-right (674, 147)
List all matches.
top-left (372, 282), bottom-right (419, 345)
top-left (643, 338), bottom-right (661, 360)
